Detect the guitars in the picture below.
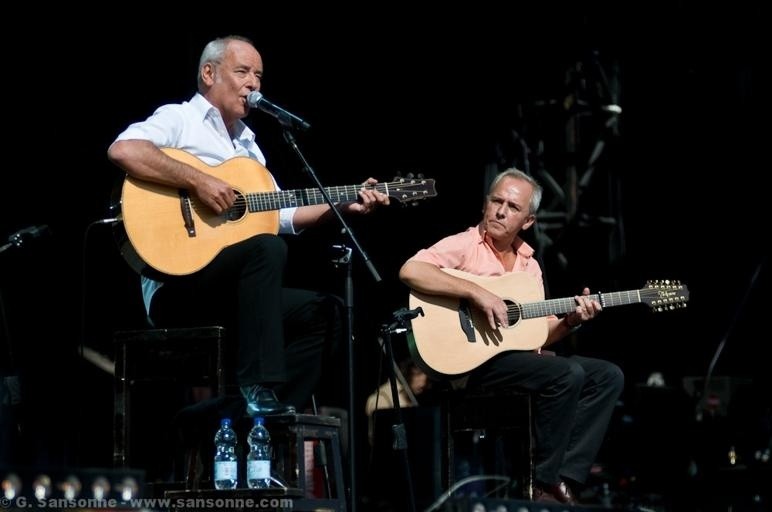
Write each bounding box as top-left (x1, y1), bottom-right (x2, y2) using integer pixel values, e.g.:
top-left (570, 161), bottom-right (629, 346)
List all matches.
top-left (118, 146), bottom-right (437, 279)
top-left (405, 260), bottom-right (690, 377)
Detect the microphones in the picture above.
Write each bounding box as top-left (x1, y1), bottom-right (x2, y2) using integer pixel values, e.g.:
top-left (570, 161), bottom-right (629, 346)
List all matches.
top-left (247, 90), bottom-right (314, 136)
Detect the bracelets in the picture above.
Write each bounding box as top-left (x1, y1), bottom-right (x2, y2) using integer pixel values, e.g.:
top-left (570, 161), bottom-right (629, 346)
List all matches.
top-left (561, 313), bottom-right (580, 329)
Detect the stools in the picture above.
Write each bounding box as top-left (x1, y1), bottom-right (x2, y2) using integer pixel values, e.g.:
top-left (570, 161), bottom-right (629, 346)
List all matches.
top-left (439, 381), bottom-right (541, 499)
top-left (264, 413), bottom-right (346, 512)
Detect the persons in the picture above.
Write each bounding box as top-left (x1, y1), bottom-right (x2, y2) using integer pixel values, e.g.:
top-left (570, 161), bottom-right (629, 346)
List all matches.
top-left (106, 34), bottom-right (391, 489)
top-left (398, 164), bottom-right (624, 511)
top-left (364, 355), bottom-right (428, 470)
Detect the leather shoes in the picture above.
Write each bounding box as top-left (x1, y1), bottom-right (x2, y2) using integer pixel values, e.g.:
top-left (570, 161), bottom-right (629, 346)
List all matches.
top-left (238, 383), bottom-right (297, 419)
top-left (535, 480), bottom-right (580, 504)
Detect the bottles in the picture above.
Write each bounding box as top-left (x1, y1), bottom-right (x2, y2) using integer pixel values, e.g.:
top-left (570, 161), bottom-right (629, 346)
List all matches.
top-left (247, 417), bottom-right (273, 490)
top-left (215, 417), bottom-right (237, 489)
